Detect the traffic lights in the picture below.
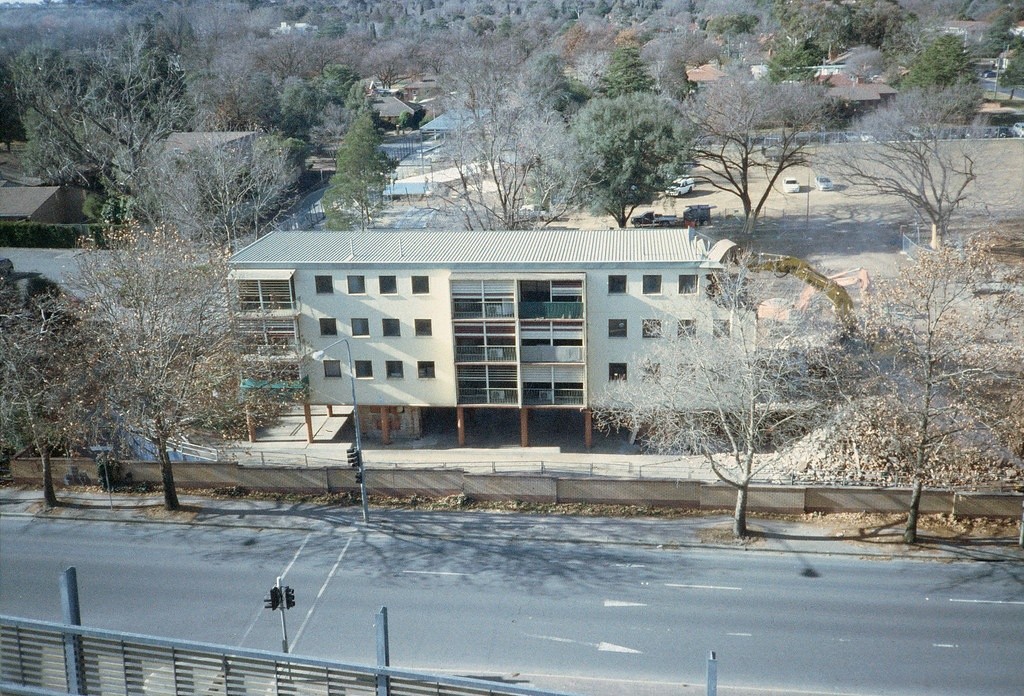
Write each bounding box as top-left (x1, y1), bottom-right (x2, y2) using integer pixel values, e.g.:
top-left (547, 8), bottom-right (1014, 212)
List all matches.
top-left (355, 472), bottom-right (363, 484)
top-left (284, 587), bottom-right (295, 609)
top-left (347, 446), bottom-right (361, 468)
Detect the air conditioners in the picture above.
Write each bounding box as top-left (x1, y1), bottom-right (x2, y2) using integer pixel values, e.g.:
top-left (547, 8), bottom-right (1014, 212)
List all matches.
top-left (491, 391), bottom-right (504, 399)
top-left (490, 349), bottom-right (503, 358)
top-left (486, 305), bottom-right (501, 315)
top-left (539, 391), bottom-right (552, 400)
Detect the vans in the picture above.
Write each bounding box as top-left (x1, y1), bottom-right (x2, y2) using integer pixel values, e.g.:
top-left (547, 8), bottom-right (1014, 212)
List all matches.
top-left (665, 177), bottom-right (698, 197)
top-left (515, 205), bottom-right (555, 221)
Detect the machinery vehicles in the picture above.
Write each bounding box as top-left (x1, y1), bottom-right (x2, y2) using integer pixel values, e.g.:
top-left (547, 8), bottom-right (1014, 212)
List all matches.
top-left (738, 250), bottom-right (871, 354)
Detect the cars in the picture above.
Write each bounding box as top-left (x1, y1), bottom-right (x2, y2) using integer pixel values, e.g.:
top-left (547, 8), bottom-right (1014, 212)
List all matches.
top-left (815, 176), bottom-right (834, 192)
top-left (783, 176), bottom-right (802, 192)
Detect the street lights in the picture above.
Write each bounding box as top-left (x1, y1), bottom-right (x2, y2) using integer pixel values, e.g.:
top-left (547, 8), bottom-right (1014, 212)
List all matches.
top-left (312, 338), bottom-right (370, 521)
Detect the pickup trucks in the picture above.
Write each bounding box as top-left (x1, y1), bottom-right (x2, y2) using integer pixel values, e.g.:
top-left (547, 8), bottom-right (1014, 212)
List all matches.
top-left (632, 212), bottom-right (676, 227)
